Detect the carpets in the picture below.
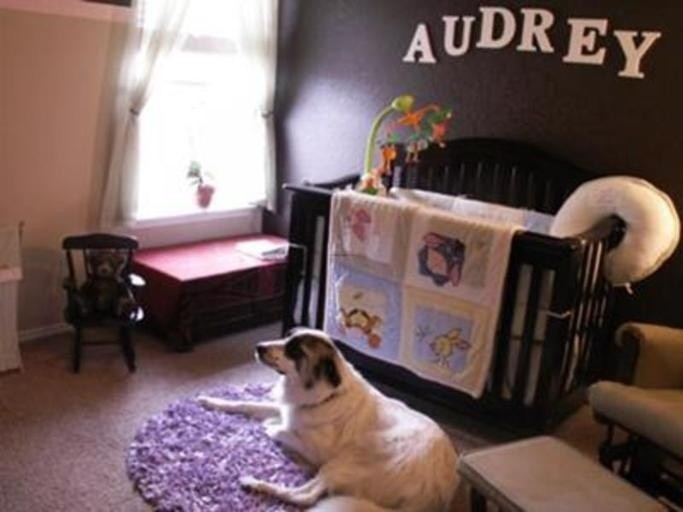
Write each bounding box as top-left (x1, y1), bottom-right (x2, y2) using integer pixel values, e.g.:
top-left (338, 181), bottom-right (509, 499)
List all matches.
top-left (124, 382), bottom-right (389, 511)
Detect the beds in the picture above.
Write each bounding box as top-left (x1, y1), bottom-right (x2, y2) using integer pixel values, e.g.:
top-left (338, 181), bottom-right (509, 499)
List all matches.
top-left (279, 135), bottom-right (619, 439)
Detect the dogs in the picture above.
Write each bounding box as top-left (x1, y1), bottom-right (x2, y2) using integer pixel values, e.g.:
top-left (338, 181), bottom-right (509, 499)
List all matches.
top-left (193, 323), bottom-right (473, 512)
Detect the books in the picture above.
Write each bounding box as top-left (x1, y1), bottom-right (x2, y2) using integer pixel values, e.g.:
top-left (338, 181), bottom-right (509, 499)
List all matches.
top-left (237, 238), bottom-right (288, 259)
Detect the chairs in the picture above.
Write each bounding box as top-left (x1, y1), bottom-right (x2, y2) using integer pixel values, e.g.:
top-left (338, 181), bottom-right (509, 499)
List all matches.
top-left (59, 231), bottom-right (144, 374)
top-left (586, 321), bottom-right (682, 511)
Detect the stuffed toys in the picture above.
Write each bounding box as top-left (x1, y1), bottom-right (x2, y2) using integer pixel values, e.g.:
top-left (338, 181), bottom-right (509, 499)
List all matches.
top-left (60, 245), bottom-right (138, 326)
